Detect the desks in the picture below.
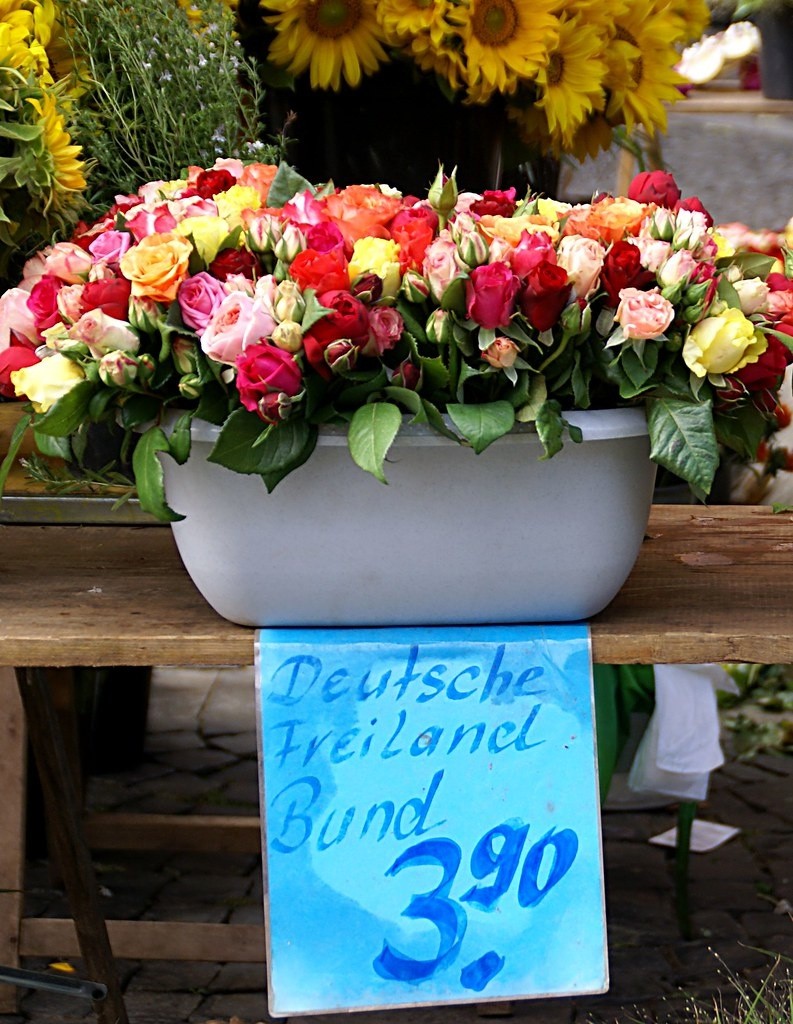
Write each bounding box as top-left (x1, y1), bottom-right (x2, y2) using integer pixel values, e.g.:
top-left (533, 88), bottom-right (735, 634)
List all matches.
top-left (0, 501), bottom-right (793, 1024)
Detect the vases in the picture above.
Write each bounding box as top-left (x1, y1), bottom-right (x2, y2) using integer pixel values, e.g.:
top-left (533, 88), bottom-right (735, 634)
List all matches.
top-left (159, 412), bottom-right (655, 623)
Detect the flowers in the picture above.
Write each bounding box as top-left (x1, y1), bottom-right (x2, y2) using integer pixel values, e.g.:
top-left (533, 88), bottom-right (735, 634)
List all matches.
top-left (0, 0), bottom-right (793, 524)
